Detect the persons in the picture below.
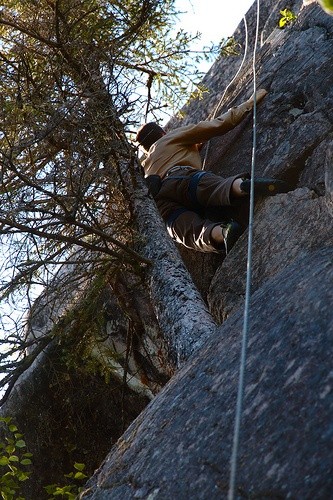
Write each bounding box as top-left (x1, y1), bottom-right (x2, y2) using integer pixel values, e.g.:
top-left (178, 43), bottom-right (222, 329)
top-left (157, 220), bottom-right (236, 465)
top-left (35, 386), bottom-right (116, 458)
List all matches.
top-left (135, 89), bottom-right (288, 257)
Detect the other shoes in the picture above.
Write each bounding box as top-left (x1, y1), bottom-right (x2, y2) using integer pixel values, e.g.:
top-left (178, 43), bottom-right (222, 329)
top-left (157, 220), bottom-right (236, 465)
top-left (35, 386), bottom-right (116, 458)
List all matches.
top-left (239, 178), bottom-right (289, 197)
top-left (219, 221), bottom-right (243, 256)
